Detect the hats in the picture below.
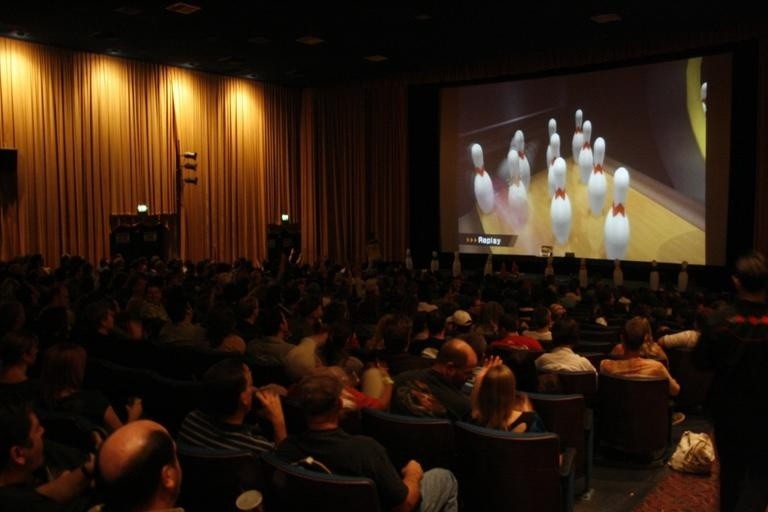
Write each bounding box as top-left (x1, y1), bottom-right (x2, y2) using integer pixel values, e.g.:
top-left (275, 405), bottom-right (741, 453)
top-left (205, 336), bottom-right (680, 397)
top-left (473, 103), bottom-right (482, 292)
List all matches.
top-left (286, 374), bottom-right (346, 417)
top-left (446, 309), bottom-right (472, 326)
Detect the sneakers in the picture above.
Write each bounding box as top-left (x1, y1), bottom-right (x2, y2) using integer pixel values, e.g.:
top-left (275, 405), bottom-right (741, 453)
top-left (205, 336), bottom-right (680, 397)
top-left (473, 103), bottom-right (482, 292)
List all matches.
top-left (671, 412), bottom-right (686, 426)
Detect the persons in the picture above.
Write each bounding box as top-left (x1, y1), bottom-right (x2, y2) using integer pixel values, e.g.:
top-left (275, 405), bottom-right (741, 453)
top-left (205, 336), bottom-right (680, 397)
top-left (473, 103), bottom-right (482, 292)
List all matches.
top-left (703, 250), bottom-right (768, 510)
top-left (1, 250), bottom-right (739, 512)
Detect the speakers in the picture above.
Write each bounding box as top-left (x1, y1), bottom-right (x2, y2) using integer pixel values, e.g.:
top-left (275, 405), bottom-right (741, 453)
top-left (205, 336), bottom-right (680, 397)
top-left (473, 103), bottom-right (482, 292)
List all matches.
top-left (0, 149), bottom-right (17, 199)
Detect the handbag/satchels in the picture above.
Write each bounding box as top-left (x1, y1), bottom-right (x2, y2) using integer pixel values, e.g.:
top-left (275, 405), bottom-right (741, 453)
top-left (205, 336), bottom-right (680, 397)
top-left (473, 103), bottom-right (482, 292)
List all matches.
top-left (668, 430), bottom-right (716, 474)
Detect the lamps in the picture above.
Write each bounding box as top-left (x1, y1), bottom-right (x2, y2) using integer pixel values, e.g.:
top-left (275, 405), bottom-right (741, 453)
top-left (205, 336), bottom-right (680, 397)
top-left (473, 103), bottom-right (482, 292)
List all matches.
top-left (174, 139), bottom-right (198, 257)
